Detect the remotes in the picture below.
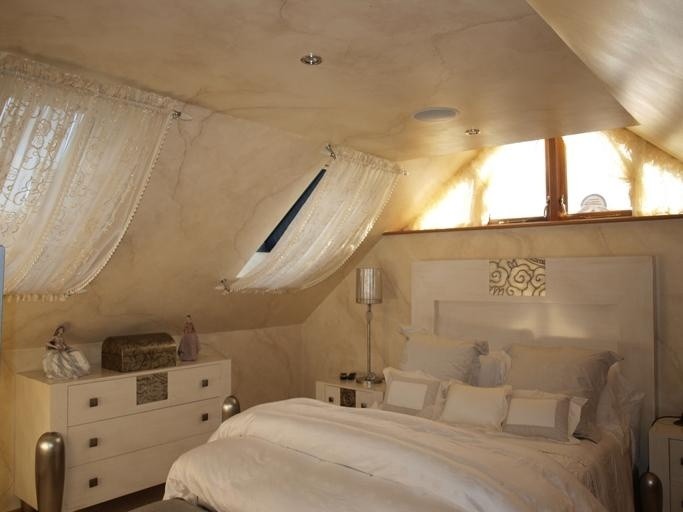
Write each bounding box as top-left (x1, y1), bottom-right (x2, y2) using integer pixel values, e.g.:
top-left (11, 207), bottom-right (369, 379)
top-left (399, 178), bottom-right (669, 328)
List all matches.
top-left (341, 373), bottom-right (347, 379)
top-left (348, 373), bottom-right (356, 380)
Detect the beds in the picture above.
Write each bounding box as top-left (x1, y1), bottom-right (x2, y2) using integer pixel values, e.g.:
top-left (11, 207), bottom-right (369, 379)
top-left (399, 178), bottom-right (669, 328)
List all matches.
top-left (162, 256), bottom-right (656, 512)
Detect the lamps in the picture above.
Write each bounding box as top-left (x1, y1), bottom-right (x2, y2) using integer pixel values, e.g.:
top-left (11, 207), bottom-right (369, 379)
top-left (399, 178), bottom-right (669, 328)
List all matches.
top-left (355, 268), bottom-right (382, 386)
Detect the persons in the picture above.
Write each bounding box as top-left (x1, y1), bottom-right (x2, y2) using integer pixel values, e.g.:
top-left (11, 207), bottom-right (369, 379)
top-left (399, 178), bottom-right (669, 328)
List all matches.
top-left (40, 325), bottom-right (91, 381)
top-left (177, 313), bottom-right (202, 362)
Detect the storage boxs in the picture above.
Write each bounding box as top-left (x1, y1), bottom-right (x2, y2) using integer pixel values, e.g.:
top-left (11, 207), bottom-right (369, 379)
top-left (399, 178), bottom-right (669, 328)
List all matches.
top-left (99, 332), bottom-right (177, 372)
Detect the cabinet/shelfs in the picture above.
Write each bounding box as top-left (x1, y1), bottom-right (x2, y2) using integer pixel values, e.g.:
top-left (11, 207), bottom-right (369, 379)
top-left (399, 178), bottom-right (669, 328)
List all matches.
top-left (14, 353), bottom-right (233, 512)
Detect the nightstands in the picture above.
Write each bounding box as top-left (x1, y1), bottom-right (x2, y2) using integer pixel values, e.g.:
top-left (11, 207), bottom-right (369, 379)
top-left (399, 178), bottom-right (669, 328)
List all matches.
top-left (314, 373), bottom-right (386, 409)
top-left (647, 416), bottom-right (681, 512)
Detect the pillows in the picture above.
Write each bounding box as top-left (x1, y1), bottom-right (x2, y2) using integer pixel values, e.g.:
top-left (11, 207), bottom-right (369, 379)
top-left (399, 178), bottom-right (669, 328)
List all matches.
top-left (439, 379), bottom-right (512, 435)
top-left (375, 366), bottom-right (443, 421)
top-left (476, 348), bottom-right (509, 387)
top-left (502, 341), bottom-right (624, 446)
top-left (594, 358), bottom-right (630, 432)
top-left (502, 389), bottom-right (588, 444)
top-left (394, 323), bottom-right (487, 386)
